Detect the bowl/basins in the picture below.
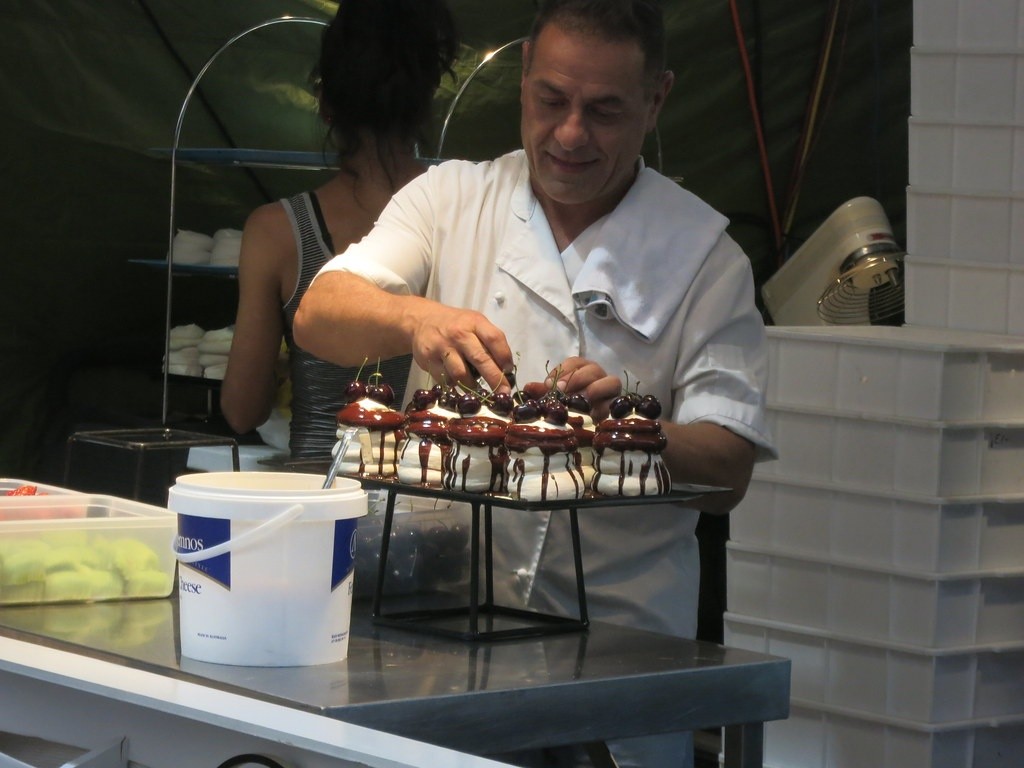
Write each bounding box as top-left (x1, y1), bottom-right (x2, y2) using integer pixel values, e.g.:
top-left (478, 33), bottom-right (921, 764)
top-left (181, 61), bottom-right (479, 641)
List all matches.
top-left (0, 478), bottom-right (82, 496)
top-left (0, 493), bottom-right (178, 606)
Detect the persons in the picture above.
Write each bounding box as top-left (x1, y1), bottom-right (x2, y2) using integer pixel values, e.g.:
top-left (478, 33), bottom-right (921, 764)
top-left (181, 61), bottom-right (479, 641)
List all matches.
top-left (220, 0), bottom-right (462, 459)
top-left (291, 0), bottom-right (780, 768)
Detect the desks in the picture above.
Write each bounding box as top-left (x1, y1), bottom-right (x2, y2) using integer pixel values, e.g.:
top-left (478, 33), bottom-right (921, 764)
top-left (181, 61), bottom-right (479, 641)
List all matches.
top-left (0, 598), bottom-right (791, 752)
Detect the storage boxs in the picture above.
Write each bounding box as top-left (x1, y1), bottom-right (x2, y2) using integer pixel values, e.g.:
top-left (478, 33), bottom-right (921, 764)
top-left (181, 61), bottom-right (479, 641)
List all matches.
top-left (0, 478), bottom-right (178, 606)
top-left (723, 326), bottom-right (1024, 768)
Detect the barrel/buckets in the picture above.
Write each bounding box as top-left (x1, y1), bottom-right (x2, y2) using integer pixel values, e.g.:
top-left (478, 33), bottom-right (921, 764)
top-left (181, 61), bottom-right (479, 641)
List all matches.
top-left (168, 472), bottom-right (369, 667)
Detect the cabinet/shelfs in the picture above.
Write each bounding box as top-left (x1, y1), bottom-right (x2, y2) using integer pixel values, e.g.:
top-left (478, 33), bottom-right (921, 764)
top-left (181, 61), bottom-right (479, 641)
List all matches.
top-left (126, 148), bottom-right (480, 446)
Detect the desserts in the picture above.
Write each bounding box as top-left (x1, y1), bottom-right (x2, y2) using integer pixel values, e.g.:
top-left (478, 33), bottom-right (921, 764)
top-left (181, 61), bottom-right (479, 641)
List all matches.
top-left (331, 356), bottom-right (671, 503)
